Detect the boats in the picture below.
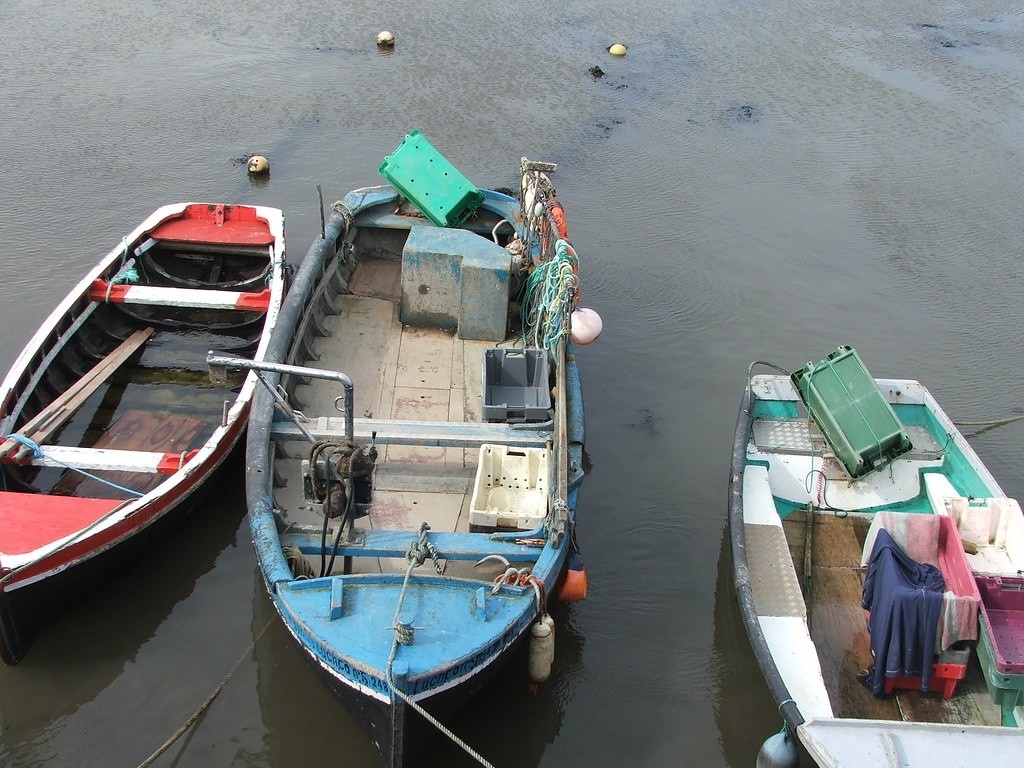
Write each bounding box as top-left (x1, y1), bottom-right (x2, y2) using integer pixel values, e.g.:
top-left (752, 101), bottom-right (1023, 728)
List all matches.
top-left (729, 343), bottom-right (1024, 768)
top-left (244, 127), bottom-right (581, 726)
top-left (1, 203), bottom-right (289, 600)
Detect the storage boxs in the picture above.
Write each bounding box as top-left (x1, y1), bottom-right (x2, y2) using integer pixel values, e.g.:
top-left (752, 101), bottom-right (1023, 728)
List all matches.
top-left (481, 347), bottom-right (551, 424)
top-left (467, 445), bottom-right (550, 534)
top-left (377, 129), bottom-right (486, 228)
top-left (791, 345), bottom-right (1024, 728)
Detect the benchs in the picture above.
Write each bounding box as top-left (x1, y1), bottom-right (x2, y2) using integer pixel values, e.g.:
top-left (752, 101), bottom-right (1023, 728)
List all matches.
top-left (271, 401), bottom-right (555, 448)
top-left (0, 436), bottom-right (200, 473)
top-left (90, 275), bottom-right (271, 312)
